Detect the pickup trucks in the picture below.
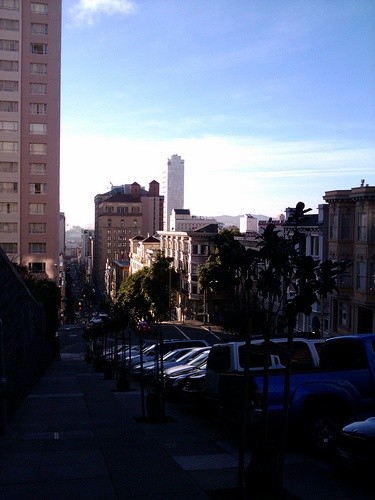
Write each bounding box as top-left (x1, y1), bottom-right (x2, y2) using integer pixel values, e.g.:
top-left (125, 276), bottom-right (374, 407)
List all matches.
top-left (217, 333), bottom-right (375, 455)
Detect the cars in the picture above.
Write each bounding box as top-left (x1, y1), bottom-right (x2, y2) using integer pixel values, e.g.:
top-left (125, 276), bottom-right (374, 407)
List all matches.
top-left (335, 410), bottom-right (375, 487)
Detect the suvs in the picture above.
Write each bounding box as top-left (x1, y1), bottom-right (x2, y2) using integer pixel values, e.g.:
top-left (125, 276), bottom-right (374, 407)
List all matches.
top-left (102, 338), bottom-right (211, 405)
top-left (206, 338), bottom-right (328, 413)
